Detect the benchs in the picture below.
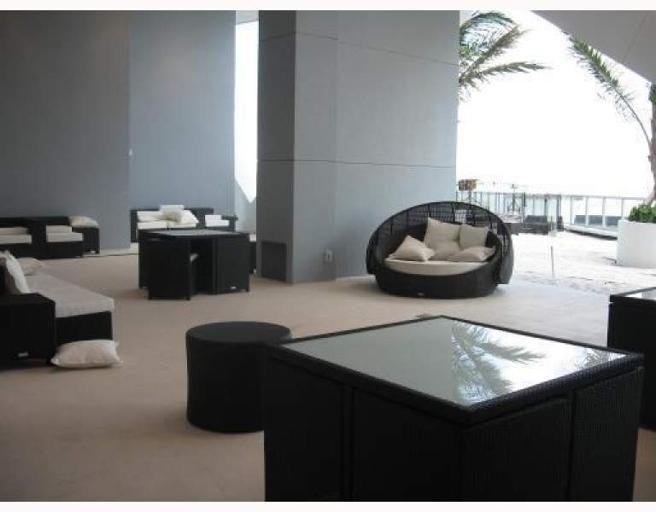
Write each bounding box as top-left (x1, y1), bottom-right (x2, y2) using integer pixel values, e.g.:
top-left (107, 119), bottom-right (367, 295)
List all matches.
top-left (568, 215), bottom-right (622, 238)
top-left (0, 207), bottom-right (238, 361)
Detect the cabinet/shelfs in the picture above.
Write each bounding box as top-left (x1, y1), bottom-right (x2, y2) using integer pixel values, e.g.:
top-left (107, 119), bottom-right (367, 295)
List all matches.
top-left (137, 229), bottom-right (251, 300)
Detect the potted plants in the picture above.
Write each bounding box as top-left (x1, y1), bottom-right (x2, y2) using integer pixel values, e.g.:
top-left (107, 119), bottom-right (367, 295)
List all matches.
top-left (615, 203), bottom-right (656, 268)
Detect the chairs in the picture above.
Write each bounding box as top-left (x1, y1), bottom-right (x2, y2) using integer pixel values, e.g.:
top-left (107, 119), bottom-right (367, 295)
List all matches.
top-left (365, 200), bottom-right (515, 297)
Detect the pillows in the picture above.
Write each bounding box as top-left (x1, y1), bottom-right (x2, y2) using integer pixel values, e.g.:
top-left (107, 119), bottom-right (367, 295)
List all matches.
top-left (52, 339), bottom-right (119, 371)
top-left (158, 204), bottom-right (185, 221)
top-left (395, 217), bottom-right (496, 262)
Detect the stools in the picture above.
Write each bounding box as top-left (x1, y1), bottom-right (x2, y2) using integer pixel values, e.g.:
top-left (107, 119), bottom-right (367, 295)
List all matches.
top-left (184, 321), bottom-right (292, 434)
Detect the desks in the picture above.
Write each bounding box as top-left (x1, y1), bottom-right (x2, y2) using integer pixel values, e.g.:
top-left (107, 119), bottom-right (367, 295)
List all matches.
top-left (263, 315), bottom-right (643, 501)
top-left (603, 286), bottom-right (656, 435)
